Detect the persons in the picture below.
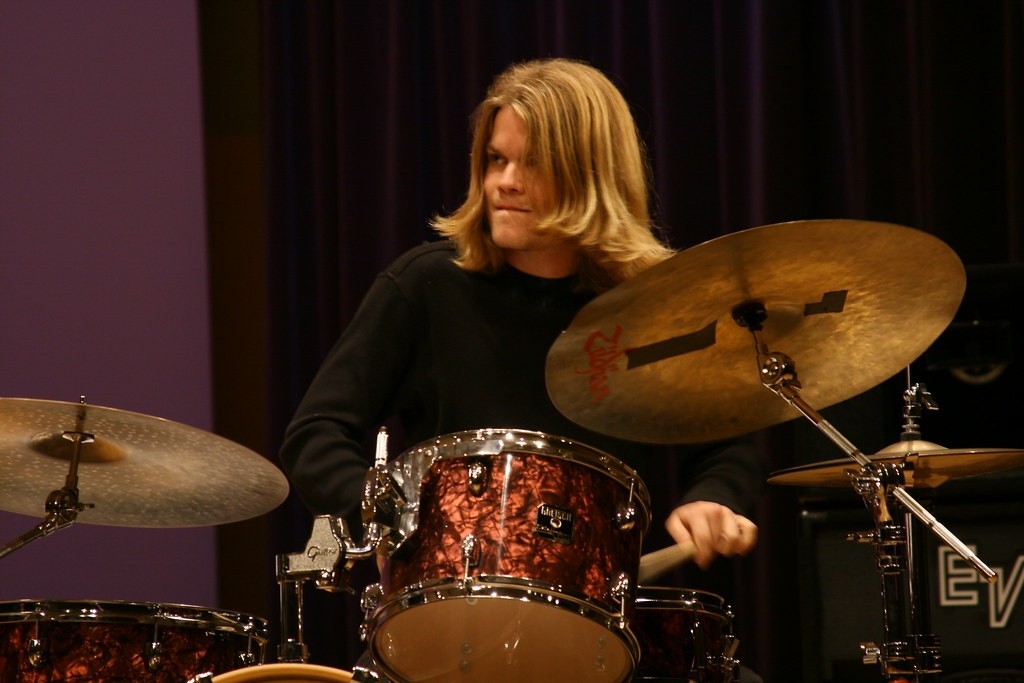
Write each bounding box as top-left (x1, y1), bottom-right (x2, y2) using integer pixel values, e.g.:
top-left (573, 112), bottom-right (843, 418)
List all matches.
top-left (280, 58), bottom-right (769, 651)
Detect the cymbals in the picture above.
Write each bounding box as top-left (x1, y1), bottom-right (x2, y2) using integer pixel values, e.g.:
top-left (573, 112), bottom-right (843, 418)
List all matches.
top-left (545, 215), bottom-right (965, 444)
top-left (0, 393), bottom-right (294, 527)
top-left (764, 440), bottom-right (1024, 489)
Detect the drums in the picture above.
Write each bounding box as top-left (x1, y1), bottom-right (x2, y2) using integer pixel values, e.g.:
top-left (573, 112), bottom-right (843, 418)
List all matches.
top-left (0, 599), bottom-right (278, 682)
top-left (630, 586), bottom-right (738, 682)
top-left (358, 430), bottom-right (646, 682)
